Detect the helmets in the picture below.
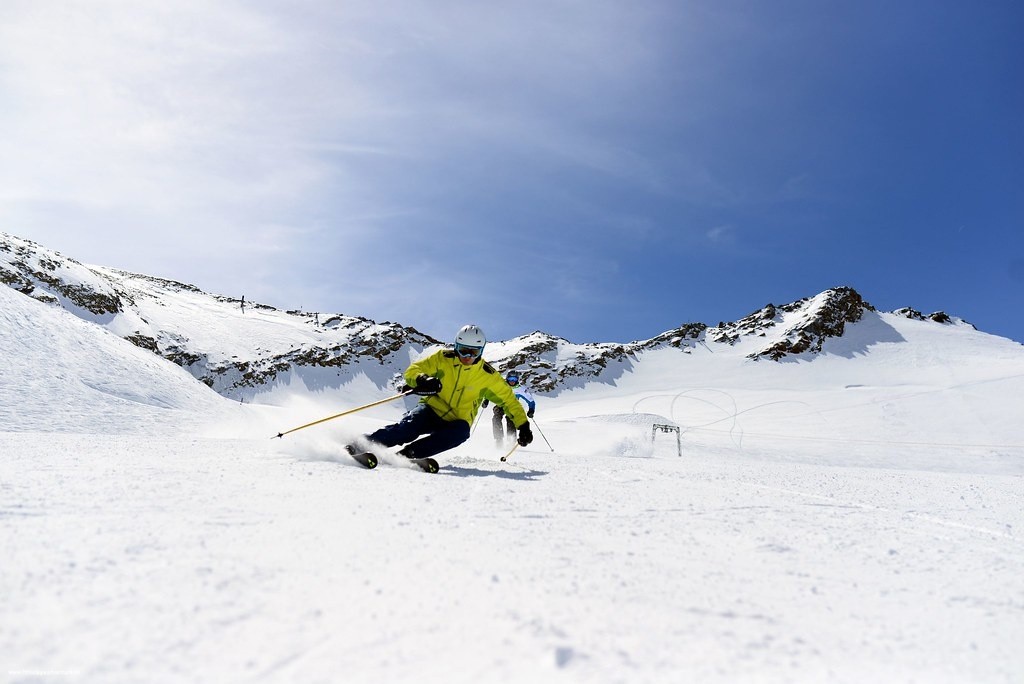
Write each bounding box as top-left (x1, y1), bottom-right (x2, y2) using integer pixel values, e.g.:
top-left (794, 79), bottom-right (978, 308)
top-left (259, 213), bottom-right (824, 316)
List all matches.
top-left (455, 324), bottom-right (486, 348)
top-left (506, 370), bottom-right (519, 378)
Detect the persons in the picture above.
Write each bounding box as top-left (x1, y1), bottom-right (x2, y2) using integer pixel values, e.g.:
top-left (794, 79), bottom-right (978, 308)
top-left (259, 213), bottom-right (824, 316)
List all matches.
top-left (350, 323), bottom-right (533, 478)
top-left (481, 367), bottom-right (539, 452)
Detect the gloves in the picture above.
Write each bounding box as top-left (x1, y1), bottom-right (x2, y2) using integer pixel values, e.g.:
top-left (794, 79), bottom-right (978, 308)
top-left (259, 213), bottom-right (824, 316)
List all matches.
top-left (526, 408), bottom-right (534, 418)
top-left (516, 422), bottom-right (534, 446)
top-left (416, 374), bottom-right (442, 393)
top-left (482, 398), bottom-right (488, 408)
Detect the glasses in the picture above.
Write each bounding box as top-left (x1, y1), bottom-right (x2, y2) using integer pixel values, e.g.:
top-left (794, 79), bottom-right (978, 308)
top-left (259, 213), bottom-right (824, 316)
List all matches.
top-left (506, 376), bottom-right (518, 381)
top-left (455, 343), bottom-right (482, 358)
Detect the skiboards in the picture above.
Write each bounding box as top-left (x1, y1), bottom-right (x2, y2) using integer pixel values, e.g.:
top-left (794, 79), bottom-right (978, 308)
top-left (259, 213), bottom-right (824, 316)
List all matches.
top-left (342, 443), bottom-right (439, 476)
